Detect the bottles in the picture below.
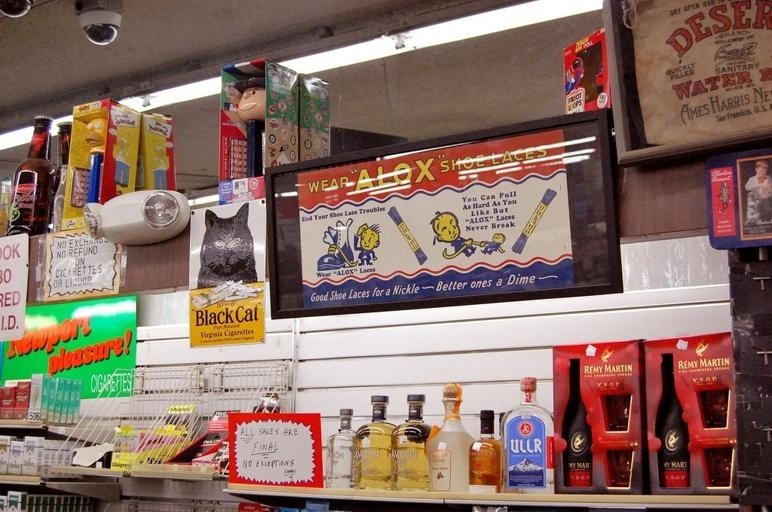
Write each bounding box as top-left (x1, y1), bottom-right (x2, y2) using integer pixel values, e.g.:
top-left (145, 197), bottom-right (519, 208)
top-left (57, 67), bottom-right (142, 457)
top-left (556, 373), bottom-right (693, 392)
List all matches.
top-left (500, 376), bottom-right (556, 494)
top-left (6, 114), bottom-right (73, 235)
top-left (325, 408), bottom-right (357, 488)
top-left (656, 353), bottom-right (691, 490)
top-left (468, 409), bottom-right (501, 492)
top-left (562, 358), bottom-right (592, 488)
top-left (391, 393), bottom-right (431, 491)
top-left (427, 382), bottom-right (476, 492)
top-left (352, 395), bottom-right (396, 490)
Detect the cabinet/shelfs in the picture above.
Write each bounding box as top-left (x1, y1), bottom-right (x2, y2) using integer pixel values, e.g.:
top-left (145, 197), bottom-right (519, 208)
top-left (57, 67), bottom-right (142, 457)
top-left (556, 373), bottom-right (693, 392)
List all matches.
top-left (0, 359), bottom-right (294, 511)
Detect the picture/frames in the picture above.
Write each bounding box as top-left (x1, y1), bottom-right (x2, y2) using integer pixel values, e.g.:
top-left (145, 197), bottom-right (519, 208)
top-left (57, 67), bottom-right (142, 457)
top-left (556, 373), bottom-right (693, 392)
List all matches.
top-left (264, 108), bottom-right (623, 320)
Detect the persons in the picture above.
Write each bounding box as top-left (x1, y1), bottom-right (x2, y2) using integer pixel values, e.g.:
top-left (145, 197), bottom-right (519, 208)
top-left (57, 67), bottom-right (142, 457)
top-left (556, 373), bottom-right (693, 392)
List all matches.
top-left (744, 161), bottom-right (772, 229)
top-left (234, 74), bottom-right (266, 177)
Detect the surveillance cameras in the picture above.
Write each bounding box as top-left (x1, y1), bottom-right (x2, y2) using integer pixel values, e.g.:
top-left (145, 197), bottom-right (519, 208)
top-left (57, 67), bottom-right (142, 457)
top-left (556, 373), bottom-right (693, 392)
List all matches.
top-left (0, 0), bottom-right (31, 19)
top-left (79, 10), bottom-right (122, 45)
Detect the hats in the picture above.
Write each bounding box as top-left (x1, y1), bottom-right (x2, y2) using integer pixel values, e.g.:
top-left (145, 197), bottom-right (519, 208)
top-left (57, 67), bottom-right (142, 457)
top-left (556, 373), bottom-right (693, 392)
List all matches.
top-left (756, 160), bottom-right (769, 169)
